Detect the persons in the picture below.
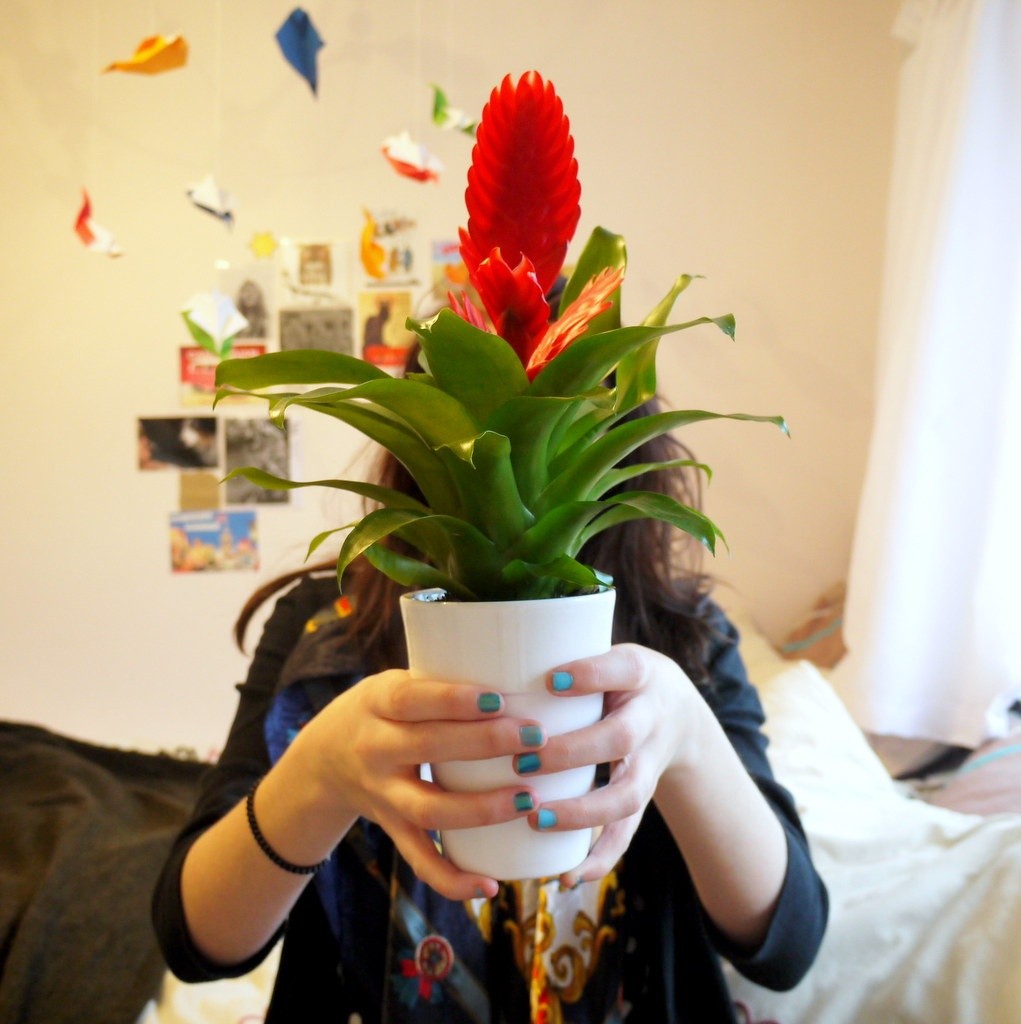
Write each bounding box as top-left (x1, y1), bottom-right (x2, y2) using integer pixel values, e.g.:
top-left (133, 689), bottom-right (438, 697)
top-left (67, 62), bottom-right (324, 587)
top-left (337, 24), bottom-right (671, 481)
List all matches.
top-left (151, 271), bottom-right (829, 1023)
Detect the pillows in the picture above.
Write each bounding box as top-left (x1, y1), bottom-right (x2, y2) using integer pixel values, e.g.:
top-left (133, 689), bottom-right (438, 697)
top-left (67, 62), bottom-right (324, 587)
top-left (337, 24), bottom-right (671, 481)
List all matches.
top-left (703, 652), bottom-right (977, 1023)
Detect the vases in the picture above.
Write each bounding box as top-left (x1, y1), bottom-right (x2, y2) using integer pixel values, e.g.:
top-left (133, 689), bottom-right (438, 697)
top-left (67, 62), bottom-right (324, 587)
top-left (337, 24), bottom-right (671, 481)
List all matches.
top-left (398, 569), bottom-right (620, 884)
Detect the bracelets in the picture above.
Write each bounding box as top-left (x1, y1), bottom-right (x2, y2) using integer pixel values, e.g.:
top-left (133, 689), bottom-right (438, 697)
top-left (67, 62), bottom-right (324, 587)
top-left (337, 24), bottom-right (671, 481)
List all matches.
top-left (247, 776), bottom-right (330, 875)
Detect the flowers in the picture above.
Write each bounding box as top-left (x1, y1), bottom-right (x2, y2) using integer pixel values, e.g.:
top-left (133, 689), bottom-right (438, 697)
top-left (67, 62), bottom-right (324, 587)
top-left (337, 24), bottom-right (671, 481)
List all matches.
top-left (203, 60), bottom-right (801, 598)
